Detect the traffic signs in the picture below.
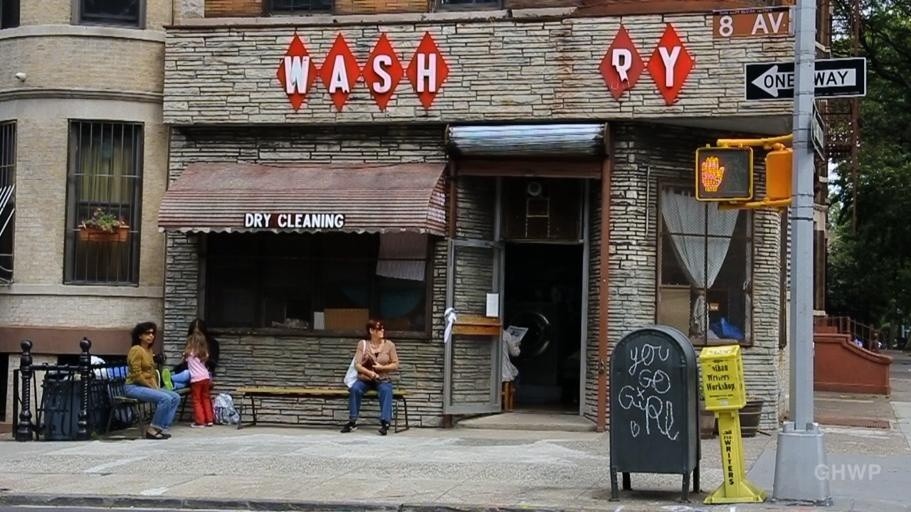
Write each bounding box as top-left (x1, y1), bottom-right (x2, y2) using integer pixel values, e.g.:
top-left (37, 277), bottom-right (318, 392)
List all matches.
top-left (745, 58), bottom-right (867, 102)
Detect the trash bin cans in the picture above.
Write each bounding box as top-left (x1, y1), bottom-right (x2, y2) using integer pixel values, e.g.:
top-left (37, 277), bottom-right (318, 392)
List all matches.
top-left (610, 325), bottom-right (702, 503)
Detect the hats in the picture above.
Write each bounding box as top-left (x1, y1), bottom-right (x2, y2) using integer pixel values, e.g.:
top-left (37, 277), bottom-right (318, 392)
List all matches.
top-left (131, 322), bottom-right (156, 349)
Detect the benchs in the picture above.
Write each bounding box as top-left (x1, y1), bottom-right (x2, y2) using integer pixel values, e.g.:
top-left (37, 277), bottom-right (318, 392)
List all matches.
top-left (103, 386), bottom-right (191, 438)
top-left (236, 385), bottom-right (410, 434)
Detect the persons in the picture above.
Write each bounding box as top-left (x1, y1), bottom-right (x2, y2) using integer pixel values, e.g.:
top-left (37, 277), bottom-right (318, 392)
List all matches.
top-left (161, 317), bottom-right (220, 391)
top-left (502, 329), bottom-right (521, 385)
top-left (340, 319), bottom-right (400, 435)
top-left (182, 331), bottom-right (214, 429)
top-left (122, 321), bottom-right (182, 440)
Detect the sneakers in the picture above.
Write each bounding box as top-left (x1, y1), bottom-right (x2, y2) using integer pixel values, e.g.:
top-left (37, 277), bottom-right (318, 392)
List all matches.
top-left (154, 368), bottom-right (175, 391)
top-left (341, 422), bottom-right (358, 433)
top-left (379, 422), bottom-right (389, 434)
top-left (146, 429), bottom-right (171, 439)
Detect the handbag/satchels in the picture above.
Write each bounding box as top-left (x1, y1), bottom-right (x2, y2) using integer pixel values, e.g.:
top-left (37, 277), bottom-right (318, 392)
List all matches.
top-left (213, 393), bottom-right (240, 425)
top-left (343, 357), bottom-right (359, 388)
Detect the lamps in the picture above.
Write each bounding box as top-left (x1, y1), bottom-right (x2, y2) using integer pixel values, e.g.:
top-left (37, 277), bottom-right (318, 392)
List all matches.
top-left (14, 72), bottom-right (27, 82)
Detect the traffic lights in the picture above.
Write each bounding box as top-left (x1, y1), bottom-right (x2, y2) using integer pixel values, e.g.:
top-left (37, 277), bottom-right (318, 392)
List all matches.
top-left (695, 134), bottom-right (792, 211)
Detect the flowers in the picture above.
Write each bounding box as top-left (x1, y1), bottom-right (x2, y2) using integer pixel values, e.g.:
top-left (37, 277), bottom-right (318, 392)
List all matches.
top-left (80, 206), bottom-right (127, 235)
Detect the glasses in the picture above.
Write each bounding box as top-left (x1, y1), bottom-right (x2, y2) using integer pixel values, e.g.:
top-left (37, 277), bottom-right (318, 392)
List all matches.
top-left (143, 331), bottom-right (155, 335)
top-left (374, 327), bottom-right (384, 331)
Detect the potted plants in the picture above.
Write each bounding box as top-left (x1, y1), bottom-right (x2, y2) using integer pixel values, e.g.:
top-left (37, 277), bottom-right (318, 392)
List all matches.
top-left (698, 382), bottom-right (716, 438)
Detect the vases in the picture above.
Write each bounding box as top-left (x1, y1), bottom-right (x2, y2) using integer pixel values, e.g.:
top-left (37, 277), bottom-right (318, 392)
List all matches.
top-left (738, 397), bottom-right (764, 437)
top-left (76, 224), bottom-right (130, 242)
